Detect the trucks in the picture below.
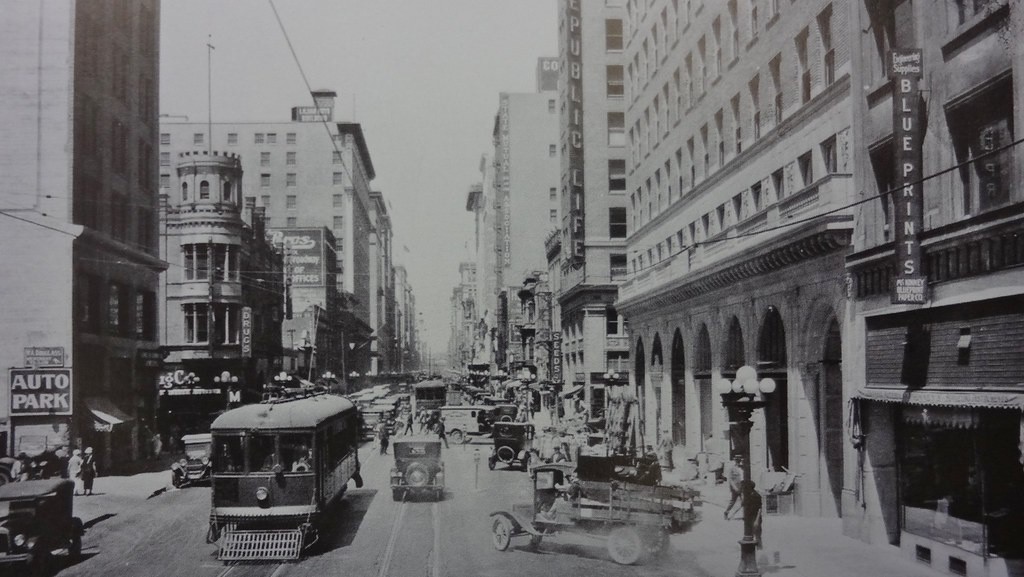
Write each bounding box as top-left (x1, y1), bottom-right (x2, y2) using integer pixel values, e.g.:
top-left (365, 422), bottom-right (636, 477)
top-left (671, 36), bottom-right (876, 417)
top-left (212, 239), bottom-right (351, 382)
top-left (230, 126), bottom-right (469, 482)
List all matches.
top-left (491, 454), bottom-right (701, 563)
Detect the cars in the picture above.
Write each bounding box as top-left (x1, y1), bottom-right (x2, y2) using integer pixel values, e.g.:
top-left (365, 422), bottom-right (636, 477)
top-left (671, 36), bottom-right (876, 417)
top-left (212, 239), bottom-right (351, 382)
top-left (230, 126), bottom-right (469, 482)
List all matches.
top-left (0, 477), bottom-right (84, 577)
top-left (171, 432), bottom-right (213, 488)
top-left (353, 361), bottom-right (534, 473)
top-left (391, 434), bottom-right (445, 500)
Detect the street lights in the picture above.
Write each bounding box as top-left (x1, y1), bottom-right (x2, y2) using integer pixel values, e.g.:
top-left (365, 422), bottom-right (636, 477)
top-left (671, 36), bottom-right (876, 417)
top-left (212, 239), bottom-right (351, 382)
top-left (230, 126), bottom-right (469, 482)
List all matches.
top-left (716, 364), bottom-right (778, 577)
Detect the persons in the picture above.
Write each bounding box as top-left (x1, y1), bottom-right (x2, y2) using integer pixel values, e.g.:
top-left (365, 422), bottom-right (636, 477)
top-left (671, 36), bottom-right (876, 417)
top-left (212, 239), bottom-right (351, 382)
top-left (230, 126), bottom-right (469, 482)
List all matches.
top-left (378, 401), bottom-right (449, 456)
top-left (528, 423), bottom-right (581, 521)
top-left (724, 455), bottom-right (762, 549)
top-left (232, 434), bottom-right (264, 474)
top-left (645, 445), bottom-right (658, 462)
top-left (557, 395), bottom-right (589, 425)
top-left (10, 438), bottom-right (98, 495)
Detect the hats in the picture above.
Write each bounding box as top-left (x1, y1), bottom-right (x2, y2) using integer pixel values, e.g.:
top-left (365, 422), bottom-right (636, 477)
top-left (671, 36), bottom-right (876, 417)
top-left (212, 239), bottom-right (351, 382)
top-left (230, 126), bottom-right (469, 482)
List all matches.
top-left (84, 447), bottom-right (93, 454)
top-left (734, 454), bottom-right (744, 459)
top-left (73, 449), bottom-right (82, 454)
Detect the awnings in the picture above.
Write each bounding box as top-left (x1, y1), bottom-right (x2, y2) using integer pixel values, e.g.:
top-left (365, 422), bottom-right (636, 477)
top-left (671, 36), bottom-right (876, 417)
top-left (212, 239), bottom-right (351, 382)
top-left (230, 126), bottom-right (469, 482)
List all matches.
top-left (346, 334), bottom-right (381, 357)
top-left (558, 385), bottom-right (584, 398)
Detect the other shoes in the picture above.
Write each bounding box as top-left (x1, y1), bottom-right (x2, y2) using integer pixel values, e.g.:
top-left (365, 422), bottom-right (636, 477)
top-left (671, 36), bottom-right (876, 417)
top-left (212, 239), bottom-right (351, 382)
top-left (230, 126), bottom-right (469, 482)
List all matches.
top-left (724, 511), bottom-right (728, 519)
top-left (75, 493), bottom-right (78, 495)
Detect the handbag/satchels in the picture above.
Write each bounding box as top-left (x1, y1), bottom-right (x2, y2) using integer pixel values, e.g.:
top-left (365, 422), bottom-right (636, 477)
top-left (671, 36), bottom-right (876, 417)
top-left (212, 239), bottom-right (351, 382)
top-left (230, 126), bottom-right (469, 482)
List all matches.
top-left (81, 471), bottom-right (84, 480)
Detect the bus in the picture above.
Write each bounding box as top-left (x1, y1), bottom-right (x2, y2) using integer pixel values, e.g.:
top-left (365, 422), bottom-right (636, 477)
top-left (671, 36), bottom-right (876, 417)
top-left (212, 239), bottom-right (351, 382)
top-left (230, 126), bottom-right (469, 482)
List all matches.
top-left (202, 376), bottom-right (361, 565)
